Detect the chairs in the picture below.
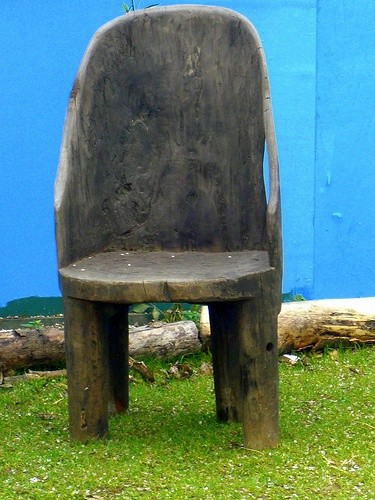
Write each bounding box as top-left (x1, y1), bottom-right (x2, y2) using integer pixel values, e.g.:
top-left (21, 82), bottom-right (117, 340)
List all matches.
top-left (53, 6), bottom-right (284, 450)
